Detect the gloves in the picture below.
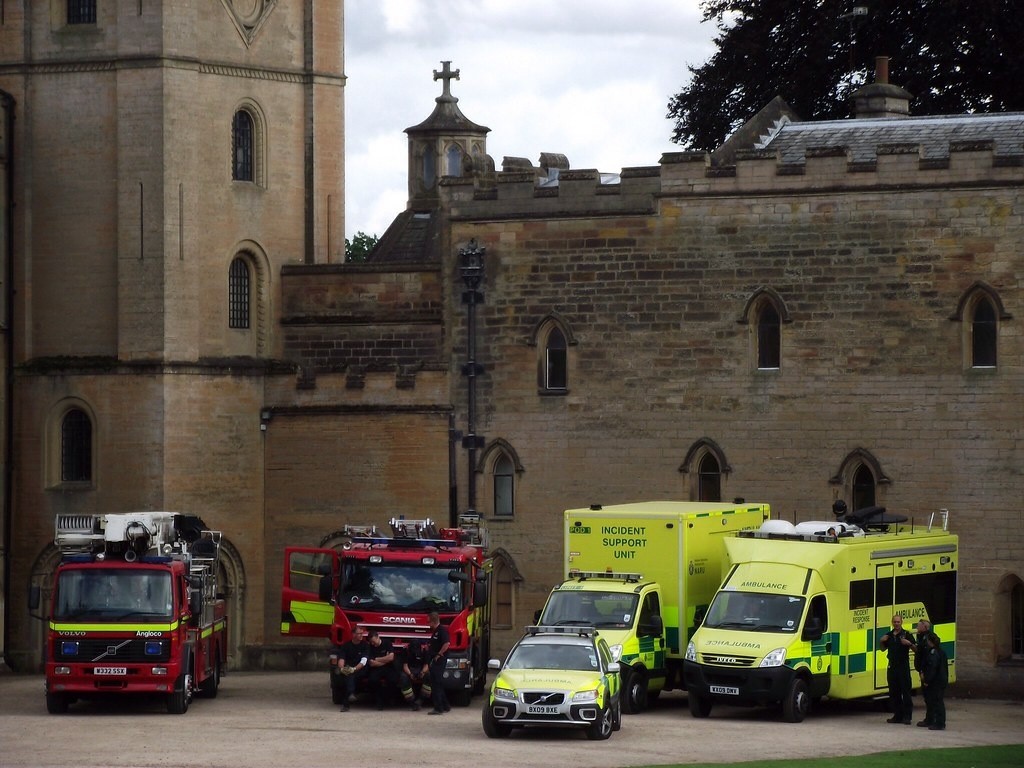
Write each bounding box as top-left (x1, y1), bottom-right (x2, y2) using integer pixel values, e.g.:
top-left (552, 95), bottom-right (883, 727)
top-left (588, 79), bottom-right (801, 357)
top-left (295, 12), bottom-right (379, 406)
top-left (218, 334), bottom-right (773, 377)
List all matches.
top-left (340, 666), bottom-right (355, 675)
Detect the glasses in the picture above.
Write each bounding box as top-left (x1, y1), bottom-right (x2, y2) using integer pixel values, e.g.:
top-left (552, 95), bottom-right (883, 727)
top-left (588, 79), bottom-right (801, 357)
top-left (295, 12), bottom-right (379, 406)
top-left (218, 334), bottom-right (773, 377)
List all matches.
top-left (919, 619), bottom-right (925, 625)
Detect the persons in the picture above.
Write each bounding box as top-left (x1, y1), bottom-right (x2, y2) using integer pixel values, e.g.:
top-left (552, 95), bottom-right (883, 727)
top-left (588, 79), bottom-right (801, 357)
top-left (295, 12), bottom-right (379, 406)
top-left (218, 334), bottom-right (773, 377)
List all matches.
top-left (336, 626), bottom-right (370, 712)
top-left (398, 640), bottom-right (431, 710)
top-left (421, 612), bottom-right (451, 715)
top-left (367, 633), bottom-right (399, 710)
top-left (923, 633), bottom-right (949, 729)
top-left (880, 614), bottom-right (916, 725)
top-left (914, 619), bottom-right (936, 726)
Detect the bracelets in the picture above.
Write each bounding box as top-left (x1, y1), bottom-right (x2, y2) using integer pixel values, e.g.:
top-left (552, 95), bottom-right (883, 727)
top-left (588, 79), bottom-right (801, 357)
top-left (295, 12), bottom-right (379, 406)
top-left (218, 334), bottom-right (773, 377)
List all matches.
top-left (910, 643), bottom-right (913, 647)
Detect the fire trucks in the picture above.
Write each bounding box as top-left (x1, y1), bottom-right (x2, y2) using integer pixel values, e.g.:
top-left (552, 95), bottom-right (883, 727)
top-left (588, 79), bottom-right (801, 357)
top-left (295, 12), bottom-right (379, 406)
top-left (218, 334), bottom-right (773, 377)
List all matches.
top-left (279, 515), bottom-right (491, 708)
top-left (26, 509), bottom-right (229, 714)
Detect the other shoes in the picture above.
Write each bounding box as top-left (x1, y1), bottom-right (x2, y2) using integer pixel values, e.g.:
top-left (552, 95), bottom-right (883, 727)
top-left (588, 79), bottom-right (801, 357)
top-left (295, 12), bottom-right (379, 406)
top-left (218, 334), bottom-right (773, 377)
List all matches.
top-left (340, 705), bottom-right (350, 712)
top-left (903, 719), bottom-right (911, 725)
top-left (349, 694), bottom-right (358, 703)
top-left (414, 698), bottom-right (425, 705)
top-left (378, 704), bottom-right (383, 710)
top-left (427, 710), bottom-right (442, 715)
top-left (887, 718), bottom-right (904, 723)
top-left (917, 721), bottom-right (929, 727)
top-left (928, 725), bottom-right (945, 730)
top-left (443, 707), bottom-right (451, 712)
top-left (412, 703), bottom-right (421, 711)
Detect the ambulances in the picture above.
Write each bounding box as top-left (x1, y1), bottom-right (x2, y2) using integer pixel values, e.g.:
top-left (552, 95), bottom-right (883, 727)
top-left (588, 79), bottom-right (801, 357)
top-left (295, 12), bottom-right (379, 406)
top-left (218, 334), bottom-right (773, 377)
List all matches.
top-left (480, 626), bottom-right (624, 738)
top-left (683, 520), bottom-right (957, 722)
top-left (534, 501), bottom-right (771, 712)
